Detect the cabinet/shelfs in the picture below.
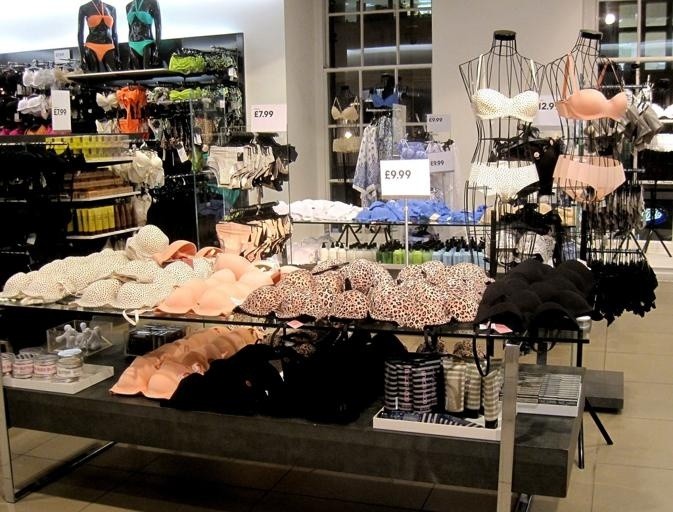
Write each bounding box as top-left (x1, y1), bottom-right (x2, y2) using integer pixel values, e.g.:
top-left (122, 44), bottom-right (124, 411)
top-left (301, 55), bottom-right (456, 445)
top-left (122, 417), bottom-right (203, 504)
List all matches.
top-left (72, 48), bottom-right (243, 255)
top-left (273, 201), bottom-right (595, 276)
top-left (0, 302), bottom-right (589, 511)
top-left (0, 60), bottom-right (143, 283)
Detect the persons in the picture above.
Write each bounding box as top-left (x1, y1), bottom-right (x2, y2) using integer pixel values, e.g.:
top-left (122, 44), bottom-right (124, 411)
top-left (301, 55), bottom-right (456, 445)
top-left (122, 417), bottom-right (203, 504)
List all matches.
top-left (459, 29), bottom-right (545, 275)
top-left (331, 86), bottom-right (360, 157)
top-left (371, 72), bottom-right (403, 125)
top-left (121, 0), bottom-right (163, 69)
top-left (542, 26), bottom-right (635, 273)
top-left (76, 0), bottom-right (122, 72)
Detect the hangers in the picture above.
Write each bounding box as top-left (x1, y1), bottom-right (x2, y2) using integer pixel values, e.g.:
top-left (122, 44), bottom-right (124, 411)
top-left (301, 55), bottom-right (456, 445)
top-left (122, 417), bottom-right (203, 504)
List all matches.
top-left (226, 200), bottom-right (282, 224)
top-left (216, 131), bottom-right (291, 148)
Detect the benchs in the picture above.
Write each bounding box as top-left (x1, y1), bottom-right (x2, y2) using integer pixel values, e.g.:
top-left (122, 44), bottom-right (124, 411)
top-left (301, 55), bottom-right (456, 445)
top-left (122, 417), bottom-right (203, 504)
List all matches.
top-left (435, 366), bottom-right (625, 469)
